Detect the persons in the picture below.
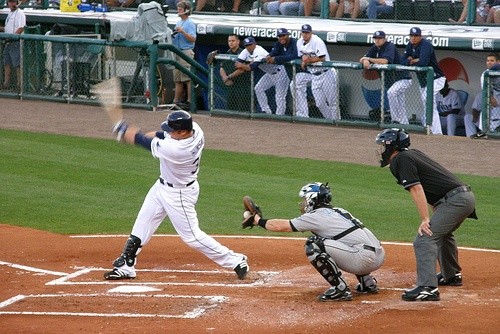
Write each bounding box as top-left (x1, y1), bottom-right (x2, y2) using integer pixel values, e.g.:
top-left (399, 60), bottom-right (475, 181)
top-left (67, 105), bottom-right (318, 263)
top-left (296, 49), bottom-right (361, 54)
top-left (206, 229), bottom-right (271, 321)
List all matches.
top-left (480, 52), bottom-right (500, 137)
top-left (173, 0), bottom-right (197, 106)
top-left (252, 182), bottom-right (385, 301)
top-left (360, 31), bottom-right (413, 125)
top-left (220, 34), bottom-right (250, 111)
top-left (329, 0), bottom-right (395, 19)
top-left (464, 91), bottom-right (482, 139)
top-left (437, 80), bottom-right (461, 136)
top-left (252, 0), bottom-right (320, 17)
top-left (375, 128), bottom-right (475, 301)
top-left (104, 110), bottom-right (250, 280)
top-left (448, 0), bottom-right (500, 24)
top-left (266, 28), bottom-right (312, 118)
top-left (296, 24), bottom-right (342, 121)
top-left (194, 0), bottom-right (242, 13)
top-left (0, 0), bottom-right (26, 90)
top-left (234, 37), bottom-right (290, 115)
top-left (399, 27), bottom-right (446, 135)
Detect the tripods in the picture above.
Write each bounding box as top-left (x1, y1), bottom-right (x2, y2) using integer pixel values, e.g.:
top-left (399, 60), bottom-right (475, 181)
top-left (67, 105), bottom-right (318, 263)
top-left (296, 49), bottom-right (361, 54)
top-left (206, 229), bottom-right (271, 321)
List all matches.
top-left (124, 55), bottom-right (160, 104)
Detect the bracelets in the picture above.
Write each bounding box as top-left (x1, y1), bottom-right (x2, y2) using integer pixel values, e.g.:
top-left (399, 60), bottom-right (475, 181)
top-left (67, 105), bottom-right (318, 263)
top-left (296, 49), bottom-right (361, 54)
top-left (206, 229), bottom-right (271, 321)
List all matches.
top-left (258, 218), bottom-right (269, 230)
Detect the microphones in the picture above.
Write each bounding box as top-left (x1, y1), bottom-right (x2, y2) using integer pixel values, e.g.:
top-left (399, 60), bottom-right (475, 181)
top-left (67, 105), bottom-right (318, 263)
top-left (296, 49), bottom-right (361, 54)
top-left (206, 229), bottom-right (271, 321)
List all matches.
top-left (14, 2), bottom-right (21, 8)
top-left (178, 10), bottom-right (186, 16)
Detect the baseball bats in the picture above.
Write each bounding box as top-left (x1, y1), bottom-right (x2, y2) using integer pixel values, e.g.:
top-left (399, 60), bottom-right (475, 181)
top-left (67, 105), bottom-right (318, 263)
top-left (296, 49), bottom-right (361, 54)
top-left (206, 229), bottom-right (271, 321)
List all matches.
top-left (93, 77), bottom-right (122, 123)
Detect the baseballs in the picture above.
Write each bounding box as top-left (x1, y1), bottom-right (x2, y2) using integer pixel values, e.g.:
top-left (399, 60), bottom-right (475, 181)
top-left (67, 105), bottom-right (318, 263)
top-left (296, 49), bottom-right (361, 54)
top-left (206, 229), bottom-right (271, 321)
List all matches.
top-left (243, 210), bottom-right (251, 219)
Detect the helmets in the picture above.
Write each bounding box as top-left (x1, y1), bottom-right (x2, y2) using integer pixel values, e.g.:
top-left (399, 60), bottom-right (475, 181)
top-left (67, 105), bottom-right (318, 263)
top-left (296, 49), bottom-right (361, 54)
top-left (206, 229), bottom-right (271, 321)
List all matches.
top-left (298, 181), bottom-right (332, 214)
top-left (161, 110), bottom-right (192, 132)
top-left (375, 128), bottom-right (410, 167)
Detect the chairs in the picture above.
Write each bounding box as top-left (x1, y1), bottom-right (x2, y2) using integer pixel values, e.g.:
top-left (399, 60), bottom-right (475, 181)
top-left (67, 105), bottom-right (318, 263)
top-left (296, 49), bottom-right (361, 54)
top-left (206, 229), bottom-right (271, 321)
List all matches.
top-left (386, 1), bottom-right (471, 24)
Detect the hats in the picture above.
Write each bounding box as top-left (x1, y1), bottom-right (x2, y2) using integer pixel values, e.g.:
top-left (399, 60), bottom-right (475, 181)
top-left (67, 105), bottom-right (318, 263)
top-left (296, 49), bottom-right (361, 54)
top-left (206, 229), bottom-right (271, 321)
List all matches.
top-left (372, 31), bottom-right (385, 38)
top-left (243, 37), bottom-right (255, 46)
top-left (277, 28), bottom-right (289, 36)
top-left (302, 24), bottom-right (311, 32)
top-left (409, 27), bottom-right (421, 36)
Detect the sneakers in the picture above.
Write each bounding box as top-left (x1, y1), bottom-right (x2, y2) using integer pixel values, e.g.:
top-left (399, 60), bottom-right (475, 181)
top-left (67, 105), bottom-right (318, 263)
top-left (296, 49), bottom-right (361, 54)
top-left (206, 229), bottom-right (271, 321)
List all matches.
top-left (317, 284), bottom-right (352, 301)
top-left (437, 272), bottom-right (462, 286)
top-left (352, 283), bottom-right (379, 294)
top-left (470, 132), bottom-right (488, 139)
top-left (234, 255), bottom-right (249, 280)
top-left (402, 286), bottom-right (440, 301)
top-left (104, 268), bottom-right (136, 280)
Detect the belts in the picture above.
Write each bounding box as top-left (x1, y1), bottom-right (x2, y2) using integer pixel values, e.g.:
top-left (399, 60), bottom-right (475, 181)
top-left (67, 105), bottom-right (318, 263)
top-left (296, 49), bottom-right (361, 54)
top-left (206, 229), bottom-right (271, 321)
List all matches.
top-left (364, 245), bottom-right (375, 251)
top-left (272, 69), bottom-right (280, 74)
top-left (313, 69), bottom-right (327, 75)
top-left (446, 186), bottom-right (471, 198)
top-left (160, 178), bottom-right (195, 187)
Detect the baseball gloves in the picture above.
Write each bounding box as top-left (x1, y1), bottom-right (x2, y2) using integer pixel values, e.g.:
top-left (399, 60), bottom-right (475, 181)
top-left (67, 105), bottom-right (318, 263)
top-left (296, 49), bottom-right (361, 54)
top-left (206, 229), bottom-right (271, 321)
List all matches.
top-left (241, 196), bottom-right (262, 229)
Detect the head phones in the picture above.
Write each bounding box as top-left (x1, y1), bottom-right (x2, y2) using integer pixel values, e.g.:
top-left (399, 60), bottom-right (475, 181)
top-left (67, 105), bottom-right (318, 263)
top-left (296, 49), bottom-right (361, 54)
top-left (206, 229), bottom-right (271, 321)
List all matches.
top-left (4, 0), bottom-right (19, 7)
top-left (184, 8), bottom-right (191, 15)
